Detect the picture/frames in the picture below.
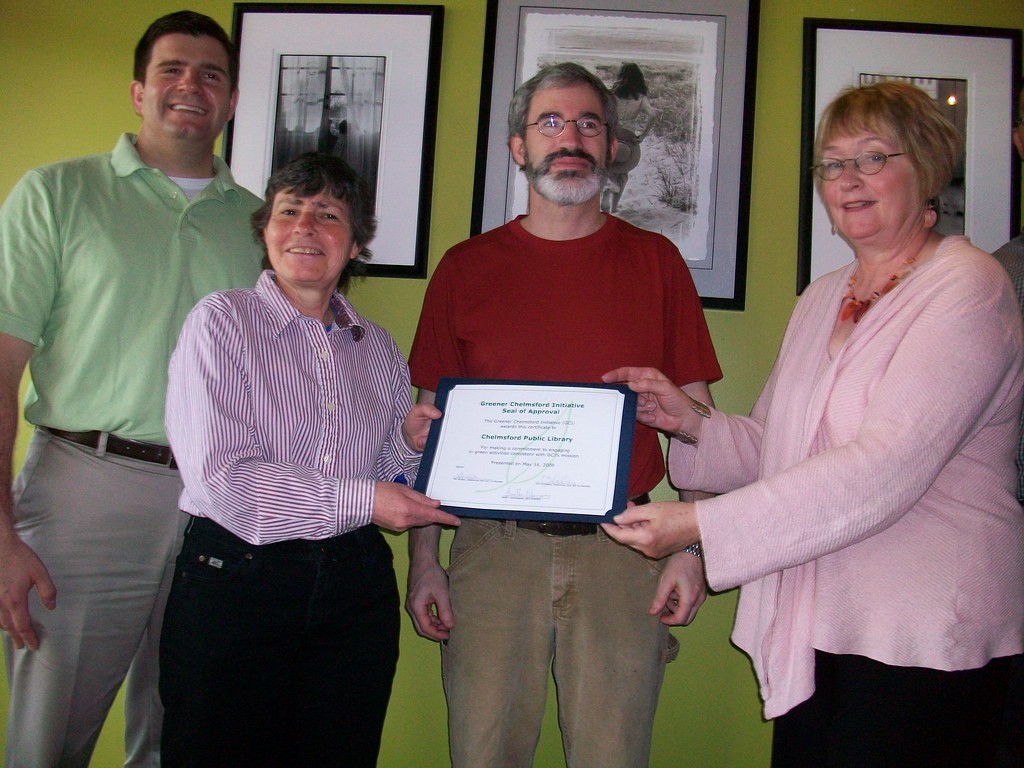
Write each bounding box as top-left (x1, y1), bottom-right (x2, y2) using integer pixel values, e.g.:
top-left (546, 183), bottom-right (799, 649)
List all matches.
top-left (796, 17), bottom-right (1024, 295)
top-left (471, 0), bottom-right (761, 312)
top-left (220, 2), bottom-right (446, 280)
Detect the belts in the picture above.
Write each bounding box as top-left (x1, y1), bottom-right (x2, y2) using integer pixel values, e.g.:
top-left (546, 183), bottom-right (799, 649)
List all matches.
top-left (47, 426), bottom-right (179, 470)
top-left (518, 491), bottom-right (651, 538)
top-left (194, 517), bottom-right (375, 560)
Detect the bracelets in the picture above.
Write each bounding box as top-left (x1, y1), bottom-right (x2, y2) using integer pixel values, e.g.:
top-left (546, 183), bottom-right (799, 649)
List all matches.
top-left (671, 400), bottom-right (712, 445)
top-left (682, 544), bottom-right (702, 558)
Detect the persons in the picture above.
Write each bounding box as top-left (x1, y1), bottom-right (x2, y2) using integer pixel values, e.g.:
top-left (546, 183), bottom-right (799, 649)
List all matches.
top-left (600, 64), bottom-right (656, 213)
top-left (601, 81), bottom-right (1023, 768)
top-left (160, 154), bottom-right (461, 768)
top-left (0, 11), bottom-right (265, 768)
top-left (404, 60), bottom-right (726, 768)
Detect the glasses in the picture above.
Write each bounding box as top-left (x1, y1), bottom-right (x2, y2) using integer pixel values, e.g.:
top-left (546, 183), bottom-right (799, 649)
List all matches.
top-left (523, 114), bottom-right (610, 137)
top-left (810, 153), bottom-right (905, 181)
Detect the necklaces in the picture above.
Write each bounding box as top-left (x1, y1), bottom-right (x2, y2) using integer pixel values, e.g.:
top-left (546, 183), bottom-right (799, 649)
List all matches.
top-left (839, 231), bottom-right (932, 324)
